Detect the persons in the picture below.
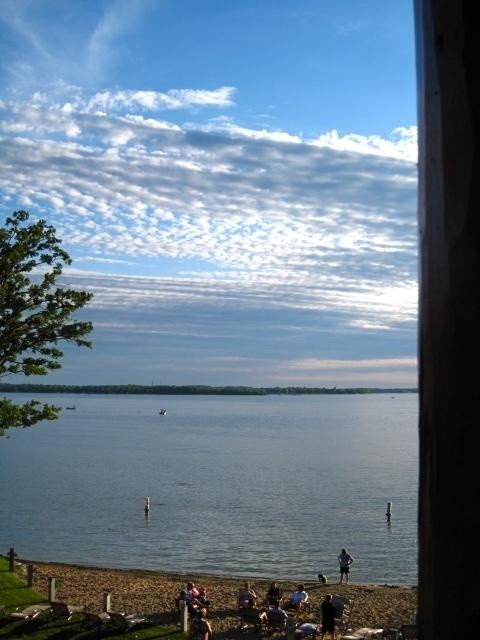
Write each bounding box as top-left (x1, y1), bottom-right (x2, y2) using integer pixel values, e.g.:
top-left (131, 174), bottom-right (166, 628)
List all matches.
top-left (318, 574), bottom-right (327, 583)
top-left (337, 548), bottom-right (354, 584)
top-left (174, 579), bottom-right (308, 639)
top-left (320, 594), bottom-right (337, 640)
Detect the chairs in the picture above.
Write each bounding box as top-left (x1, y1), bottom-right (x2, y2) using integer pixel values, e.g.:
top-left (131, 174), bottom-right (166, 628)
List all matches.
top-left (0, 596), bottom-right (152, 640)
top-left (167, 588), bottom-right (358, 640)
